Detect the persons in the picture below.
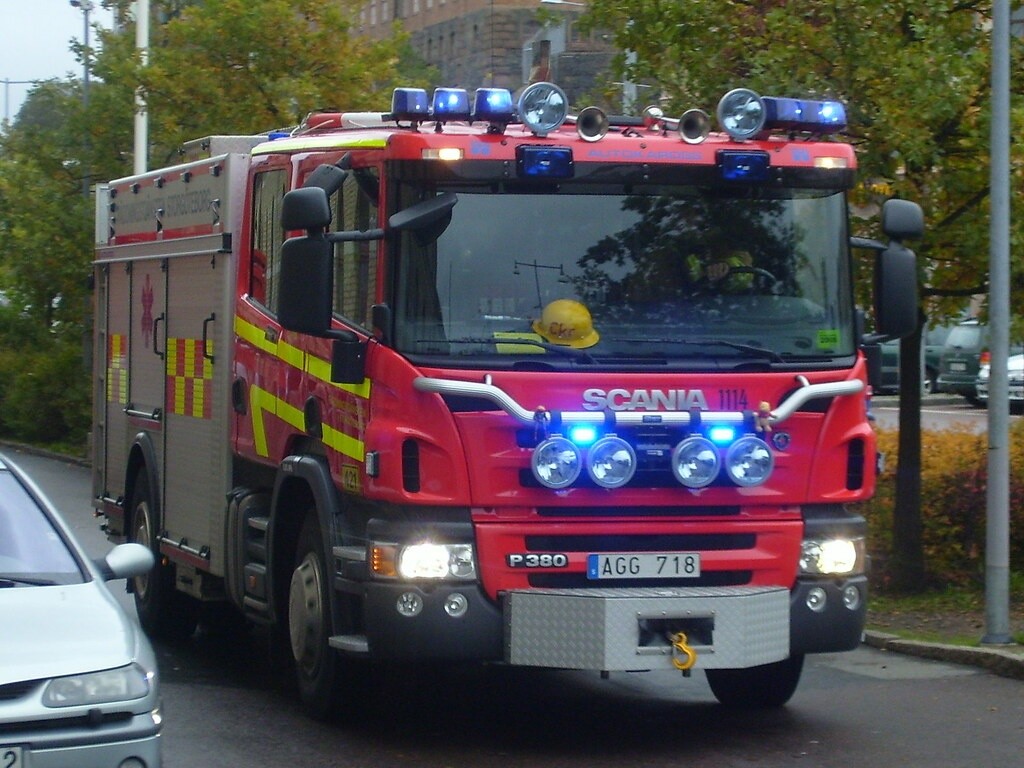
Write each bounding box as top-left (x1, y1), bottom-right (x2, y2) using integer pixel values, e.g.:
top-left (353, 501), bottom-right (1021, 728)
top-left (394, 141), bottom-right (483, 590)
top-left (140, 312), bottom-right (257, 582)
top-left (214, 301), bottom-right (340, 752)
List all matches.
top-left (665, 211), bottom-right (754, 292)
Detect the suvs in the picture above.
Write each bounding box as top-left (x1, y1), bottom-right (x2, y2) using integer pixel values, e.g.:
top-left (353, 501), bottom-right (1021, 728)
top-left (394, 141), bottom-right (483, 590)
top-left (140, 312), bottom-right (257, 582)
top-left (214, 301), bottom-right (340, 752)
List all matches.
top-left (934, 319), bottom-right (1024, 406)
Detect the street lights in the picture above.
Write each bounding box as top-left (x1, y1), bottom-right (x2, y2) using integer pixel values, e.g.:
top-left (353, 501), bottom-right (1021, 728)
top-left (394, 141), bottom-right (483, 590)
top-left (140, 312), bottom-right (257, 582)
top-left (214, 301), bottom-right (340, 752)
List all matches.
top-left (66, 0), bottom-right (94, 207)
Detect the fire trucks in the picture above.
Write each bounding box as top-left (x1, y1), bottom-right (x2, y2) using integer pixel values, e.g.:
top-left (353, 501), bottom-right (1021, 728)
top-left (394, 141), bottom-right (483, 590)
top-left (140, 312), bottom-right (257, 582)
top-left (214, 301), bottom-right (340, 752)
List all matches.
top-left (87, 84), bottom-right (922, 728)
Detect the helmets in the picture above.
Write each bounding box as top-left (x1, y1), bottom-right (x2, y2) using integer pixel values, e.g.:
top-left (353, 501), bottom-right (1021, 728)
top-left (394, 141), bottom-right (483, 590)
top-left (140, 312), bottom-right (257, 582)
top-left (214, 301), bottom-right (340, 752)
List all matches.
top-left (532, 299), bottom-right (601, 349)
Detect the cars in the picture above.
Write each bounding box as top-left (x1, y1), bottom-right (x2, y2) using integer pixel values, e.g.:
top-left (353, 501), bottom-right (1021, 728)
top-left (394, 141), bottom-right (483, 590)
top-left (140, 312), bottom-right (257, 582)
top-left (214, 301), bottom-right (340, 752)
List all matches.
top-left (975, 353), bottom-right (1023, 412)
top-left (0, 448), bottom-right (167, 768)
top-left (875, 320), bottom-right (956, 396)
